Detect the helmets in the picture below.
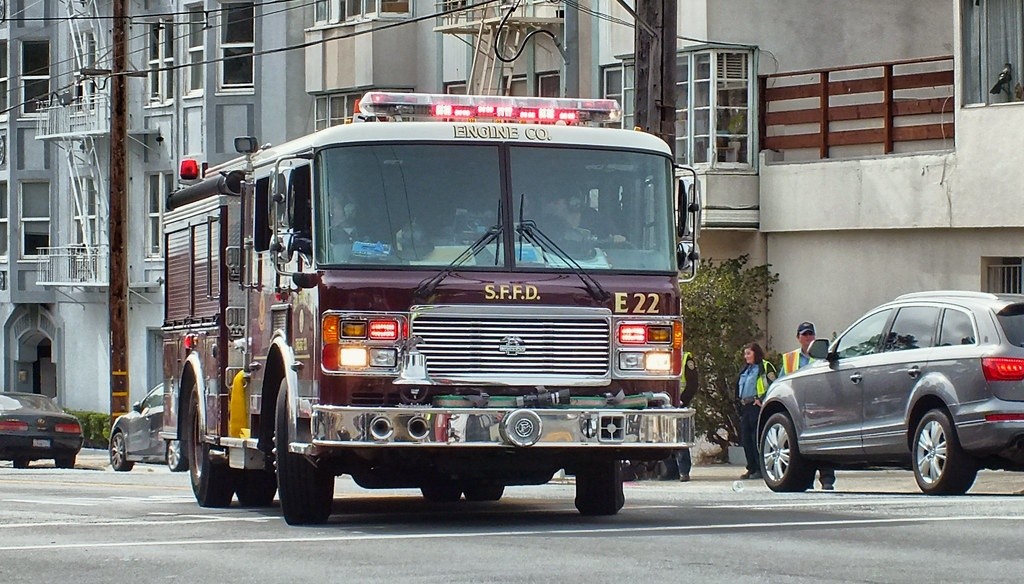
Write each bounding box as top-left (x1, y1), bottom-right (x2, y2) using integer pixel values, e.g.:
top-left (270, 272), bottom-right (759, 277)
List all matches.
top-left (393, 226), bottom-right (437, 261)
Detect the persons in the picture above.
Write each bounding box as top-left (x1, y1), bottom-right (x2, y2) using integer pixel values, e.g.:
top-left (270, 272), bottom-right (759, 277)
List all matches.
top-left (734, 343), bottom-right (781, 478)
top-left (778, 321), bottom-right (835, 489)
top-left (658, 331), bottom-right (698, 482)
top-left (537, 172), bottom-right (629, 247)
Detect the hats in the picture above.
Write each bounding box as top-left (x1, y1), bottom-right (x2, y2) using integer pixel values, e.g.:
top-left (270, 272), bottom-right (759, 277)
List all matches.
top-left (797, 321), bottom-right (815, 334)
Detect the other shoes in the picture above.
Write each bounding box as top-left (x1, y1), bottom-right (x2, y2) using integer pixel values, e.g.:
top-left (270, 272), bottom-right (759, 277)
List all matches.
top-left (804, 484), bottom-right (815, 492)
top-left (657, 469), bottom-right (681, 481)
top-left (740, 472), bottom-right (751, 480)
top-left (821, 483), bottom-right (835, 490)
top-left (749, 469), bottom-right (764, 479)
top-left (680, 473), bottom-right (690, 481)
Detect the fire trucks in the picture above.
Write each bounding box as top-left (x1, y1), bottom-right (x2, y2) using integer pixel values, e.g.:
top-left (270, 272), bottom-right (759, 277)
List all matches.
top-left (162, 90), bottom-right (702, 528)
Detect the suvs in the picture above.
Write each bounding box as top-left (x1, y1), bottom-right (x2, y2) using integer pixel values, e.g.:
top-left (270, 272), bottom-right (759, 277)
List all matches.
top-left (756, 290), bottom-right (1024, 498)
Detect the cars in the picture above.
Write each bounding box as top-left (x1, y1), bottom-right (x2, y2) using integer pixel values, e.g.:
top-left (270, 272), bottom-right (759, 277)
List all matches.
top-left (0, 391), bottom-right (85, 469)
top-left (108, 384), bottom-right (190, 473)
